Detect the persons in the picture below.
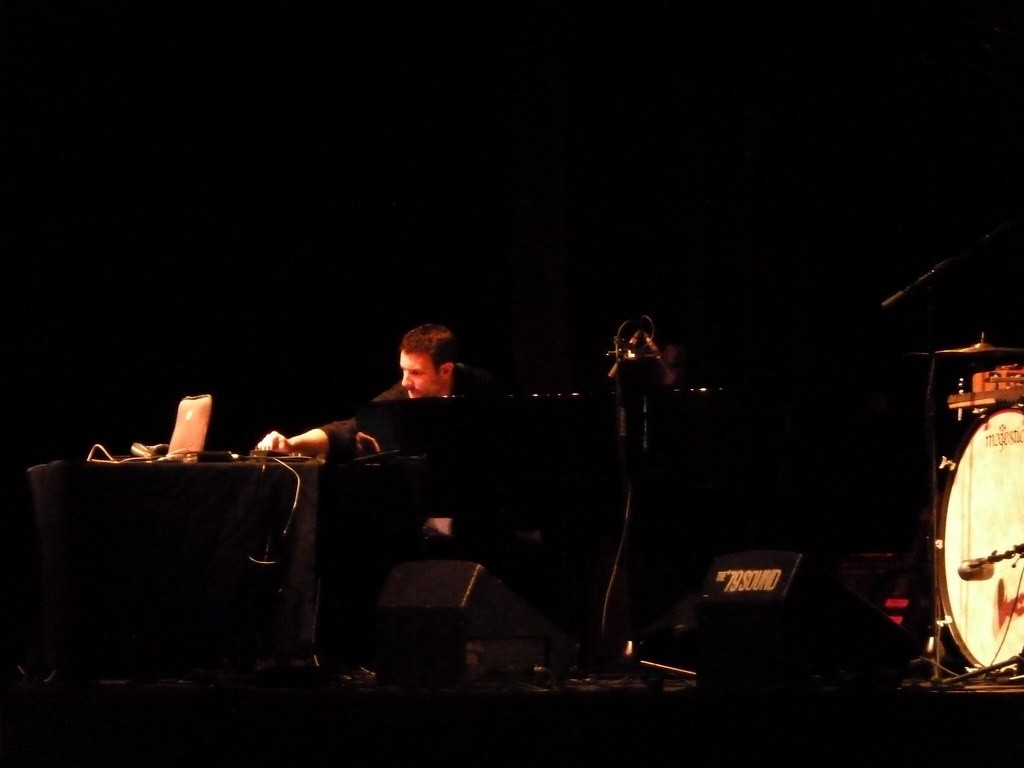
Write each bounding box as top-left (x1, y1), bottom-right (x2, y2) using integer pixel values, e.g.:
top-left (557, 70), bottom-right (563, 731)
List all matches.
top-left (255, 324), bottom-right (492, 454)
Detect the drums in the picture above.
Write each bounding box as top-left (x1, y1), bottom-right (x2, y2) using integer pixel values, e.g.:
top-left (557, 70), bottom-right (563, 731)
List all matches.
top-left (972, 370), bottom-right (1024, 394)
top-left (926, 404), bottom-right (1024, 678)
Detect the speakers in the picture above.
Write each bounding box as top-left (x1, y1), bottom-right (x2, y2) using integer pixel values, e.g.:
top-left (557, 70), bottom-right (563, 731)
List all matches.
top-left (692, 551), bottom-right (975, 672)
top-left (373, 558), bottom-right (583, 683)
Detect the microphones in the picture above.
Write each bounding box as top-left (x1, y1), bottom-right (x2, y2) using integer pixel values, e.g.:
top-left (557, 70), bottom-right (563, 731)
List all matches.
top-left (642, 332), bottom-right (675, 384)
top-left (608, 330), bottom-right (641, 378)
top-left (959, 558), bottom-right (995, 581)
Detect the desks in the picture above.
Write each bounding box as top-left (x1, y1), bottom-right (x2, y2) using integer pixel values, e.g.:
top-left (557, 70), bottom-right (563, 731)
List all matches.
top-left (27, 458), bottom-right (320, 685)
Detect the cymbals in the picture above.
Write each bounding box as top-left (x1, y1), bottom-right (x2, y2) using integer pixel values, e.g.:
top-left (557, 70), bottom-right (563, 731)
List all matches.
top-left (903, 343), bottom-right (1024, 358)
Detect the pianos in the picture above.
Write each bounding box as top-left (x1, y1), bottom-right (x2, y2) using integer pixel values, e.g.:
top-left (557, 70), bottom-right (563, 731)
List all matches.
top-left (352, 386), bottom-right (729, 682)
